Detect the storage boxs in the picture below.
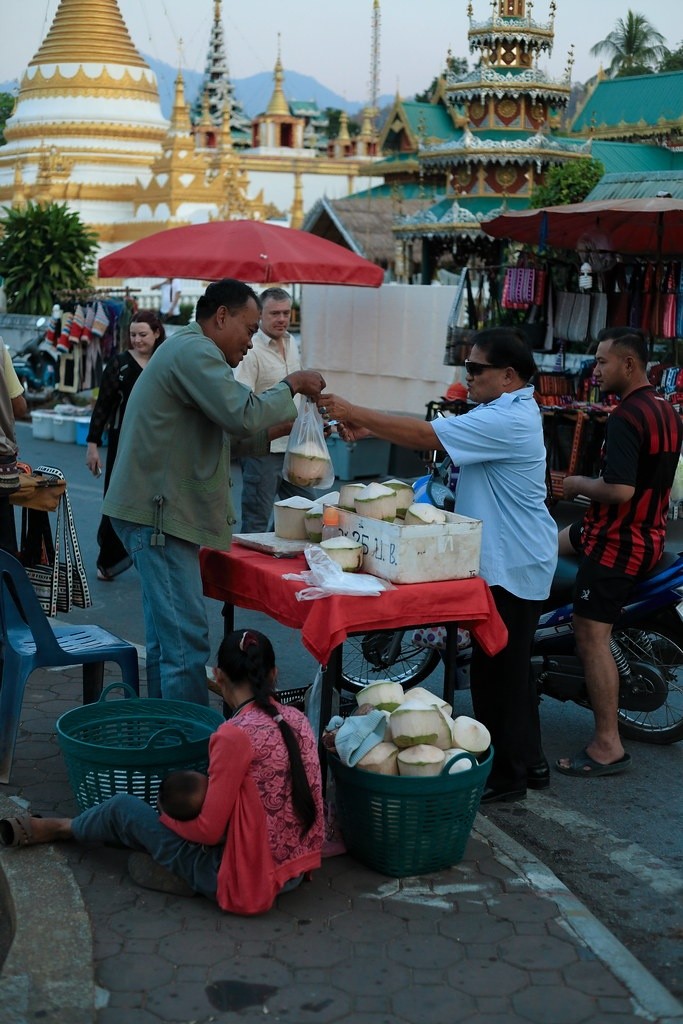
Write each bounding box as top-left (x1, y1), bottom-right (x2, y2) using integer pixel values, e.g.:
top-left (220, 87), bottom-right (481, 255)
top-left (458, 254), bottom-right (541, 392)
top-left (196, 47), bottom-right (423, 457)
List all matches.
top-left (323, 503), bottom-right (483, 581)
top-left (74, 419), bottom-right (108, 447)
top-left (392, 446), bottom-right (430, 478)
top-left (52, 416), bottom-right (75, 443)
top-left (223, 681), bottom-right (359, 719)
top-left (327, 433), bottom-right (389, 482)
top-left (30, 409), bottom-right (53, 441)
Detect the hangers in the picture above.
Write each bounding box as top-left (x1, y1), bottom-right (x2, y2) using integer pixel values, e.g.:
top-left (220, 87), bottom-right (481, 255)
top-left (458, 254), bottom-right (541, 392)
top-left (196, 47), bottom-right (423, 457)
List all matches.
top-left (56, 289), bottom-right (114, 302)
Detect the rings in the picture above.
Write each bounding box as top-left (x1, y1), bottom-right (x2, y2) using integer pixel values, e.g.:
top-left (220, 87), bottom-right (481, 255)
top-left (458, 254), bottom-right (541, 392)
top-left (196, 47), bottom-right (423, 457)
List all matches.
top-left (323, 414), bottom-right (330, 421)
top-left (319, 406), bottom-right (326, 414)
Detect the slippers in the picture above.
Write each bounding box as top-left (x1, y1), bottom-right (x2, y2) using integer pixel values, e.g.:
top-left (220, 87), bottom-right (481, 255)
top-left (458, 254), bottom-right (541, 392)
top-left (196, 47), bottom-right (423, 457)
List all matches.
top-left (0, 813), bottom-right (44, 849)
top-left (554, 746), bottom-right (632, 777)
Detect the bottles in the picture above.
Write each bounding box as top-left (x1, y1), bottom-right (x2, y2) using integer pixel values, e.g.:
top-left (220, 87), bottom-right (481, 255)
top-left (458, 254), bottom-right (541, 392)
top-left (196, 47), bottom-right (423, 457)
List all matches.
top-left (322, 507), bottom-right (340, 542)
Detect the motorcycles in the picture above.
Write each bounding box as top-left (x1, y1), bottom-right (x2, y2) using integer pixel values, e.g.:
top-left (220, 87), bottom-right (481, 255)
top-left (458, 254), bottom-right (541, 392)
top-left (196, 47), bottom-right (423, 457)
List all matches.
top-left (337, 399), bottom-right (683, 745)
top-left (4, 317), bottom-right (62, 405)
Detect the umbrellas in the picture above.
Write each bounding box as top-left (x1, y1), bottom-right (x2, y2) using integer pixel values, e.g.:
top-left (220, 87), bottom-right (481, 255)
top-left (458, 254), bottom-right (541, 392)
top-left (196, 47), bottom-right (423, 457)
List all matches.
top-left (99, 219), bottom-right (384, 289)
top-left (479, 191), bottom-right (683, 332)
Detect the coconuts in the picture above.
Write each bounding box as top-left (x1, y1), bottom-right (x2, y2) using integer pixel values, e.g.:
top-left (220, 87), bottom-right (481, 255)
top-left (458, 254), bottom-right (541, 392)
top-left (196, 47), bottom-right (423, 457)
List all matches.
top-left (353, 679), bottom-right (490, 778)
top-left (285, 441), bottom-right (331, 487)
top-left (274, 478), bottom-right (446, 573)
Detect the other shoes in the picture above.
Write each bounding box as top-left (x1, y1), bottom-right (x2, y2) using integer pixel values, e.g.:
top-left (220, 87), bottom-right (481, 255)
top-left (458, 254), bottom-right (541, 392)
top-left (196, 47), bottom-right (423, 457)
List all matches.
top-left (529, 759), bottom-right (551, 793)
top-left (480, 784), bottom-right (527, 805)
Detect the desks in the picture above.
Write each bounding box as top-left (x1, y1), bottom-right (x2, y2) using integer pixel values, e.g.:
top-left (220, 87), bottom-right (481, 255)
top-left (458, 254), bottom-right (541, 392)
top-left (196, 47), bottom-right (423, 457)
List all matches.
top-left (198, 542), bottom-right (510, 839)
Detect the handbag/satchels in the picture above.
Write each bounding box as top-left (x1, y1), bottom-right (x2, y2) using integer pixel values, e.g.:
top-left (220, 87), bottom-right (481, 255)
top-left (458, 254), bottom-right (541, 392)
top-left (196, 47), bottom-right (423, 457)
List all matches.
top-left (280, 389), bottom-right (335, 491)
top-left (0, 461), bottom-right (22, 498)
top-left (398, 244), bottom-right (683, 522)
top-left (19, 465), bottom-right (93, 617)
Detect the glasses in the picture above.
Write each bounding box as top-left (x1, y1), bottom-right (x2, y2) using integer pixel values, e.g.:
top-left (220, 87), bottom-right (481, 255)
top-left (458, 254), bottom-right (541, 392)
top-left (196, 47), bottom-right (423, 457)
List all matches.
top-left (464, 358), bottom-right (523, 377)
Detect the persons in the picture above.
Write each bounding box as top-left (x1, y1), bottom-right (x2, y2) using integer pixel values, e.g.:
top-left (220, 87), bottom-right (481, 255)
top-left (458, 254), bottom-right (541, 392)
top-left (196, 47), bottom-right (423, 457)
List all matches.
top-left (158, 769), bottom-right (229, 845)
top-left (99, 278), bottom-right (326, 706)
top-left (152, 278), bottom-right (182, 323)
top-left (0, 292), bottom-right (26, 556)
top-left (234, 287), bottom-right (302, 533)
top-left (0, 629), bottom-right (326, 916)
top-left (85, 313), bottom-right (166, 580)
top-left (552, 327), bottom-right (683, 774)
top-left (309, 331), bottom-right (559, 806)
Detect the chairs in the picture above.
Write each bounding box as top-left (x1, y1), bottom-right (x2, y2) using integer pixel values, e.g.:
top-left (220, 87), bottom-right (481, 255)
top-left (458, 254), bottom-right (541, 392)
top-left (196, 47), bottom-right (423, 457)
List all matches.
top-left (0, 549), bottom-right (141, 789)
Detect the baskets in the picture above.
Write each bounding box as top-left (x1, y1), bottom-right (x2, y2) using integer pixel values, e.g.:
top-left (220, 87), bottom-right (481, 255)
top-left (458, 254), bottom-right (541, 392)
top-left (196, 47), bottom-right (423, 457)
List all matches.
top-left (57, 681), bottom-right (226, 816)
top-left (326, 706), bottom-right (494, 880)
